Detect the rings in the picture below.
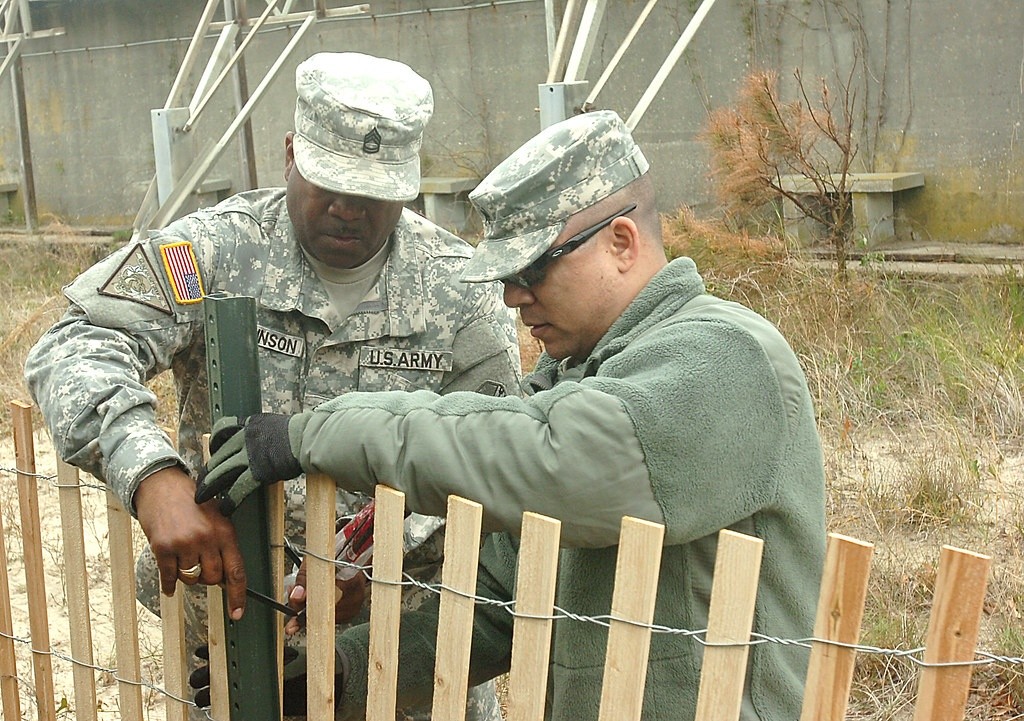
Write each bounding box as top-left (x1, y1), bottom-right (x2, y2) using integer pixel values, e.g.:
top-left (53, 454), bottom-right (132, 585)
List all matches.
top-left (178, 563), bottom-right (202, 579)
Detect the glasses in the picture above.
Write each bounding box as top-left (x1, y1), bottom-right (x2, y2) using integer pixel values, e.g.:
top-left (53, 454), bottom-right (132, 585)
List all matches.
top-left (498, 205), bottom-right (637, 289)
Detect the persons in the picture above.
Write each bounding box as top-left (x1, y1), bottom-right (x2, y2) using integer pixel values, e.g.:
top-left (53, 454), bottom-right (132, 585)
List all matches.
top-left (189, 108), bottom-right (830, 721)
top-left (22, 50), bottom-right (525, 721)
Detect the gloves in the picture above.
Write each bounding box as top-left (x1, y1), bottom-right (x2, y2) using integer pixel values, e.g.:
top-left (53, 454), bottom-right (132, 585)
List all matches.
top-left (195, 414), bottom-right (306, 517)
top-left (189, 641), bottom-right (346, 716)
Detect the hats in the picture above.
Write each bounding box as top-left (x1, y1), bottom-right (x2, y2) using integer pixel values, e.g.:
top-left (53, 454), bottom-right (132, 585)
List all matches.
top-left (293, 51), bottom-right (432, 202)
top-left (458, 109), bottom-right (650, 283)
